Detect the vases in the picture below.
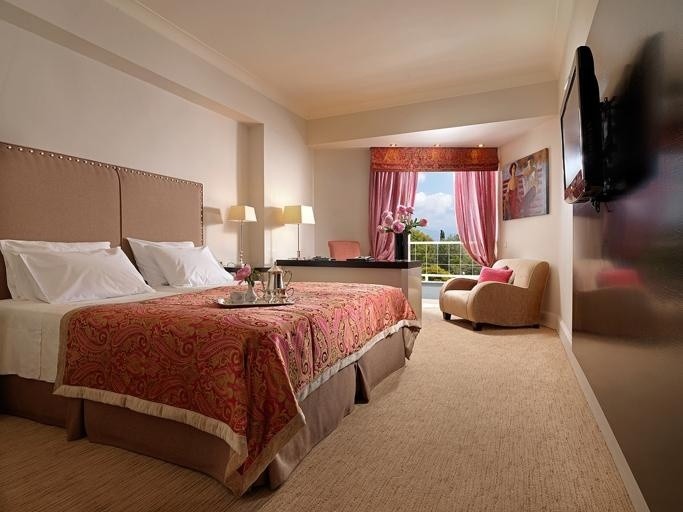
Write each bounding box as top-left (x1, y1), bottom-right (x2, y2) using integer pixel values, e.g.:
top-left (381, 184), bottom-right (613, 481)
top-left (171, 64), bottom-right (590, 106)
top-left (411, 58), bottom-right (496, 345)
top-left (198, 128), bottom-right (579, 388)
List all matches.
top-left (393, 232), bottom-right (408, 260)
top-left (244, 285), bottom-right (258, 302)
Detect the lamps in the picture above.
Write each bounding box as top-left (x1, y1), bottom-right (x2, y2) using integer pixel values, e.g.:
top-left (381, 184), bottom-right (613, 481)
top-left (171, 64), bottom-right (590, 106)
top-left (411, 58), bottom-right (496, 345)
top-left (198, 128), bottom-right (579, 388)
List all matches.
top-left (224, 204), bottom-right (258, 266)
top-left (281, 205), bottom-right (315, 261)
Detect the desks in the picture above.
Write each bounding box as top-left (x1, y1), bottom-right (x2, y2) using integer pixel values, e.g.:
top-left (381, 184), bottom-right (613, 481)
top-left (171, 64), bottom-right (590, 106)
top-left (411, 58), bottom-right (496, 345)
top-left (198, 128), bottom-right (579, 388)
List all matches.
top-left (226, 265), bottom-right (282, 289)
top-left (277, 256), bottom-right (421, 332)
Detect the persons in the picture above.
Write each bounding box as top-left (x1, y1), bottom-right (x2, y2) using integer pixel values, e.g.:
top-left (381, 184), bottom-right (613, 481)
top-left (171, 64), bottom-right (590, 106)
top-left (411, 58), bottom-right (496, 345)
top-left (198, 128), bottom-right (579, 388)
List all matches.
top-left (521, 155), bottom-right (538, 217)
top-left (503, 162), bottom-right (523, 219)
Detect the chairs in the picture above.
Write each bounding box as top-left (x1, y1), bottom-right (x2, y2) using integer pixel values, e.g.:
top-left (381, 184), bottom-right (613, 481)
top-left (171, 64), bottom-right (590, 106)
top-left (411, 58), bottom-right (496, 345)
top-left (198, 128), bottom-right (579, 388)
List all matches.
top-left (438, 257), bottom-right (549, 333)
top-left (327, 240), bottom-right (362, 261)
top-left (573, 257), bottom-right (675, 341)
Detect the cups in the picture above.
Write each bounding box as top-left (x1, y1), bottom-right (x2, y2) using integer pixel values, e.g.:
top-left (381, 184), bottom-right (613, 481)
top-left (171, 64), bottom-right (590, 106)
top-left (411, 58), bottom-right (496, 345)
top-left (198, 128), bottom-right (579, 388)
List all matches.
top-left (229, 291), bottom-right (244, 303)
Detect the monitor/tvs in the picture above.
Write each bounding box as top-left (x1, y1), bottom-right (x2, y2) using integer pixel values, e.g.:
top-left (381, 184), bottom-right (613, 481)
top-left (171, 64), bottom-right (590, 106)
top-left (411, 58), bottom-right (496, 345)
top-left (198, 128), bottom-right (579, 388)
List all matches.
top-left (558, 46), bottom-right (612, 209)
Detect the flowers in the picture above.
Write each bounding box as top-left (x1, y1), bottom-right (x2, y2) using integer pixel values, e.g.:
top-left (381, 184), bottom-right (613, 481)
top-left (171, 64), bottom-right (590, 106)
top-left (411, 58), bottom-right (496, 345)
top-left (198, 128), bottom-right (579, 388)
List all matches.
top-left (376, 204), bottom-right (428, 238)
top-left (225, 258), bottom-right (262, 288)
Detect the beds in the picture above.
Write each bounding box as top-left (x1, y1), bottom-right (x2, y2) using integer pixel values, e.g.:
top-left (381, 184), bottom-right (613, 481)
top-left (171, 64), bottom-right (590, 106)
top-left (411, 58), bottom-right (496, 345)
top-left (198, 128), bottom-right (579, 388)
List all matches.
top-left (1, 140), bottom-right (415, 502)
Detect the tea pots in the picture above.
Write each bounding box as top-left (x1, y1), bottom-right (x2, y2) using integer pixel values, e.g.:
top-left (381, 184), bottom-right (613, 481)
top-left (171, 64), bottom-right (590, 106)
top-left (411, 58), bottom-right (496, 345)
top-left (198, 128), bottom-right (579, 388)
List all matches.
top-left (259, 260), bottom-right (294, 303)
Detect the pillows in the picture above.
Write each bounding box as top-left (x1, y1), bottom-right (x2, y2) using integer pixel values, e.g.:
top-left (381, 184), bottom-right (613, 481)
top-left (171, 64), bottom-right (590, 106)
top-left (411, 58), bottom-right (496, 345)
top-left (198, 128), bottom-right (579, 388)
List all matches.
top-left (477, 265), bottom-right (513, 286)
top-left (152, 245), bottom-right (235, 288)
top-left (502, 265), bottom-right (508, 270)
top-left (9, 240), bottom-right (154, 308)
top-left (0, 234), bottom-right (111, 304)
top-left (125, 236), bottom-right (196, 288)
top-left (595, 266), bottom-right (641, 292)
